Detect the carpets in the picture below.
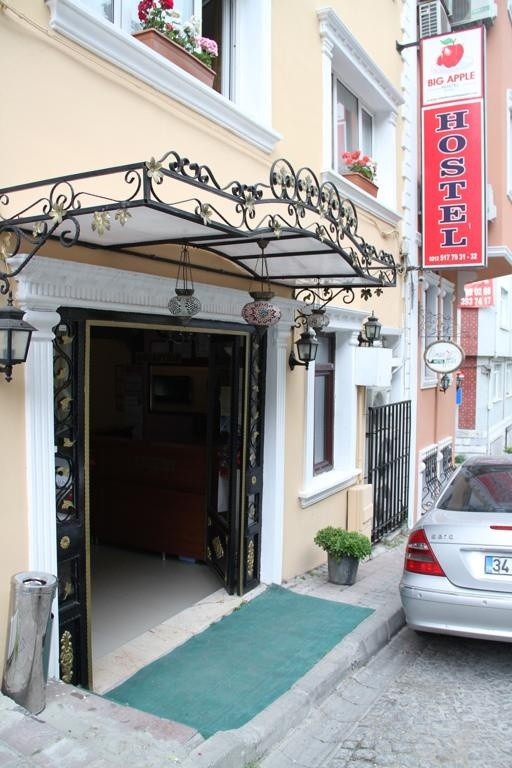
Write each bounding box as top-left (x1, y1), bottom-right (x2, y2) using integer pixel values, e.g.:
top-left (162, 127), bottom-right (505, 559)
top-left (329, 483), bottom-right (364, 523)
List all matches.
top-left (80, 580), bottom-right (376, 743)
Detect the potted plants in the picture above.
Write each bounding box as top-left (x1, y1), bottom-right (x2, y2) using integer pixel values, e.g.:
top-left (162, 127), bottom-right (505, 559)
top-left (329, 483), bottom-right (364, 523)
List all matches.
top-left (312, 524), bottom-right (376, 586)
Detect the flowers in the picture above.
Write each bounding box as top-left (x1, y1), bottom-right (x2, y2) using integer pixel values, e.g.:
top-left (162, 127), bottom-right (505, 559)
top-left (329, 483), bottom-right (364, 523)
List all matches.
top-left (137, 0), bottom-right (220, 69)
top-left (342, 148), bottom-right (378, 182)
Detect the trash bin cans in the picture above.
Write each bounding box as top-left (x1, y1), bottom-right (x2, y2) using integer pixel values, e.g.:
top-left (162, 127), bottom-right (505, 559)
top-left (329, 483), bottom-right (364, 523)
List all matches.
top-left (2, 572), bottom-right (58, 715)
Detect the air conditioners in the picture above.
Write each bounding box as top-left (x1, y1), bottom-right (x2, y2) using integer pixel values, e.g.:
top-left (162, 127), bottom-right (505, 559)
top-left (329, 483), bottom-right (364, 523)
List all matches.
top-left (418, 0), bottom-right (496, 39)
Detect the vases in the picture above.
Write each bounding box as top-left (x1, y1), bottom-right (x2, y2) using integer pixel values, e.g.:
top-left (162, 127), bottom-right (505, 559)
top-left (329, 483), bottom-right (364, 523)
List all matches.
top-left (132, 28), bottom-right (217, 91)
top-left (342, 172), bottom-right (378, 198)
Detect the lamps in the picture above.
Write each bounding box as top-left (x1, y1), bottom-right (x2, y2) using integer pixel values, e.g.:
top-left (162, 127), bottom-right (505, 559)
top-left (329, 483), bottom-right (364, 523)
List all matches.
top-left (309, 277), bottom-right (331, 337)
top-left (241, 238), bottom-right (282, 342)
top-left (356, 309), bottom-right (382, 347)
top-left (290, 323), bottom-right (319, 372)
top-left (166, 238), bottom-right (202, 328)
top-left (0, 291), bottom-right (39, 383)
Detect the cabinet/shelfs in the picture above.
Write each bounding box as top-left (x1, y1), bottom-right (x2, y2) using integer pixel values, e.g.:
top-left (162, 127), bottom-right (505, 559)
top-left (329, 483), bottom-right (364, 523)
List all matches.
top-left (91, 435), bottom-right (207, 565)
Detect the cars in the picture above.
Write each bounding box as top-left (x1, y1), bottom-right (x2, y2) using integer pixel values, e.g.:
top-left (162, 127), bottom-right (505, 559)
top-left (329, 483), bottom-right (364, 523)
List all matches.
top-left (398, 454), bottom-right (512, 644)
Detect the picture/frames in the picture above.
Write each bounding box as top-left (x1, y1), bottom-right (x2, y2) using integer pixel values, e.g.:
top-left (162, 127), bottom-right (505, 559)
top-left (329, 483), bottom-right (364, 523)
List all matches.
top-left (146, 362), bottom-right (209, 416)
